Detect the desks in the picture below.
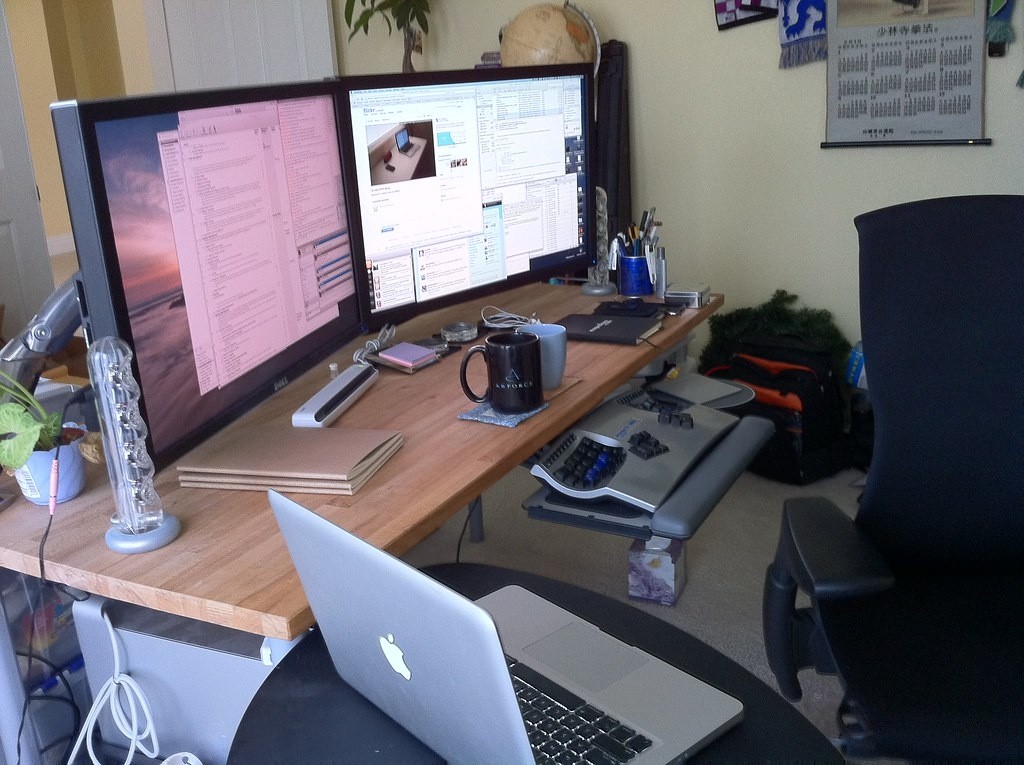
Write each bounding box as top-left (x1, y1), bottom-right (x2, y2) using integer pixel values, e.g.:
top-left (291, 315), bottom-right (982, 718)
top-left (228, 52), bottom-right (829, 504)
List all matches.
top-left (222, 561), bottom-right (850, 765)
top-left (0, 262), bottom-right (729, 764)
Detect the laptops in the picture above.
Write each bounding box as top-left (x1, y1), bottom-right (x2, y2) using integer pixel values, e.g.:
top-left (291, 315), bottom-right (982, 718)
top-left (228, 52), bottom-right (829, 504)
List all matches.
top-left (269, 489), bottom-right (742, 765)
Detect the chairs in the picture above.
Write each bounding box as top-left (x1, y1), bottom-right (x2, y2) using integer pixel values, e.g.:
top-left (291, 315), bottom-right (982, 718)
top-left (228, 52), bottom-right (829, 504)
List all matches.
top-left (760, 194), bottom-right (1024, 765)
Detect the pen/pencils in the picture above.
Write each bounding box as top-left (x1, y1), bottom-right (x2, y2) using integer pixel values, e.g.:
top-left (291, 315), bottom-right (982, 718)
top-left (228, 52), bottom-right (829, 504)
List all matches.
top-left (615, 206), bottom-right (663, 256)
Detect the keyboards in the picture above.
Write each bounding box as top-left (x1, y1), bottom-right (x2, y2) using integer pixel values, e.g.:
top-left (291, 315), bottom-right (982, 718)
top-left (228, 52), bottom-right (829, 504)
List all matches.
top-left (528, 388), bottom-right (740, 516)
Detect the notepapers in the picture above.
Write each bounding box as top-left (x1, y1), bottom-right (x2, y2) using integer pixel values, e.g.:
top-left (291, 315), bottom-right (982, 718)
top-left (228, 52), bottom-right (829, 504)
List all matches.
top-left (378, 342), bottom-right (436, 369)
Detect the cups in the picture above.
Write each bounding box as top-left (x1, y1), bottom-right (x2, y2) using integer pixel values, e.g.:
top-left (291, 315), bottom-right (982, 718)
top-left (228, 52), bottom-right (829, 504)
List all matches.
top-left (513, 324), bottom-right (568, 390)
top-left (460, 332), bottom-right (543, 415)
top-left (617, 254), bottom-right (655, 298)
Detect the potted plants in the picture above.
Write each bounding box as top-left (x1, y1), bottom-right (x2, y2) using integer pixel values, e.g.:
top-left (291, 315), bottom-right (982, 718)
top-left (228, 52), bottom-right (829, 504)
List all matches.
top-left (0, 367), bottom-right (89, 505)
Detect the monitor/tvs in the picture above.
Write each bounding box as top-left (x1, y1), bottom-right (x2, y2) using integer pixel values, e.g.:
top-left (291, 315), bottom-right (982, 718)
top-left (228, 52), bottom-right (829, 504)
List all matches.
top-left (324, 62), bottom-right (596, 333)
top-left (51, 80), bottom-right (367, 477)
